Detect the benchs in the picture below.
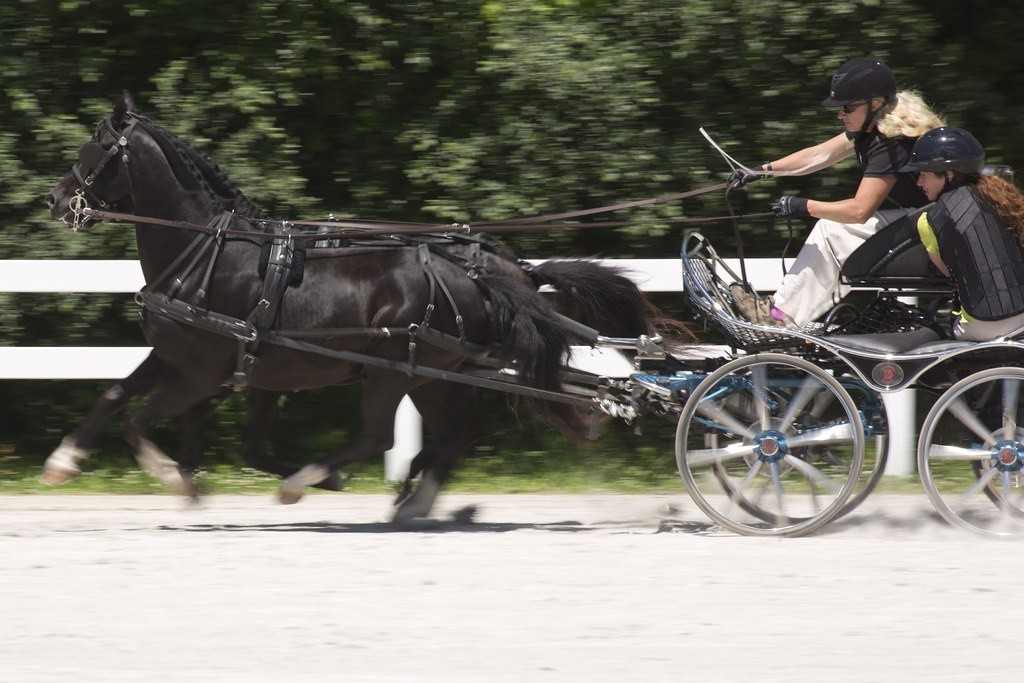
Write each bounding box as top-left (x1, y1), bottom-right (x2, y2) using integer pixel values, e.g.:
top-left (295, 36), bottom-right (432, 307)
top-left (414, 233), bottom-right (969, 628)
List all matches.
top-left (822, 164), bottom-right (1013, 340)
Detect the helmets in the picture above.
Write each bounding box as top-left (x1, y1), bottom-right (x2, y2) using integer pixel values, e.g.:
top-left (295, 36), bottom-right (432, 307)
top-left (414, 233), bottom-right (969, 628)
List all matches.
top-left (897, 126), bottom-right (986, 174)
top-left (820, 56), bottom-right (897, 107)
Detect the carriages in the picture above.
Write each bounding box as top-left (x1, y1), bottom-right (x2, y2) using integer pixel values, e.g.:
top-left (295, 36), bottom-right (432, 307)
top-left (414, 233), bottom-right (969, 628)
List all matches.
top-left (44, 87), bottom-right (1024, 537)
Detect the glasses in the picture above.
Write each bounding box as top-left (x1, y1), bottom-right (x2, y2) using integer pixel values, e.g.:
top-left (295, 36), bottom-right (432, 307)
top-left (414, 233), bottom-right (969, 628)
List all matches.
top-left (840, 102), bottom-right (870, 115)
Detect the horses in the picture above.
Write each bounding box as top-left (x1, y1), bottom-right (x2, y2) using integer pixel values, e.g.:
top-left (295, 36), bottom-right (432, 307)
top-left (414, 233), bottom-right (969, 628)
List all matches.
top-left (39, 86), bottom-right (716, 522)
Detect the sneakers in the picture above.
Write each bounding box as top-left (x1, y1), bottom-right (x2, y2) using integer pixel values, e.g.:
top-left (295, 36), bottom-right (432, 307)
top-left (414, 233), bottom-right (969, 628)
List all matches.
top-left (728, 282), bottom-right (785, 329)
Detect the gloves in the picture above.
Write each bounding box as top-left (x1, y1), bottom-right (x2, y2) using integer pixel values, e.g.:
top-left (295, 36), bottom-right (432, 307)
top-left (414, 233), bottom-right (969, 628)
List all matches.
top-left (769, 195), bottom-right (811, 219)
top-left (726, 166), bottom-right (765, 189)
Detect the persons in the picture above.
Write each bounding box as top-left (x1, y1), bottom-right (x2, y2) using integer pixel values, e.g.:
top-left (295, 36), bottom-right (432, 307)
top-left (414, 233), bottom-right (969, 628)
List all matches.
top-left (725, 56), bottom-right (946, 332)
top-left (897, 127), bottom-right (1024, 341)
